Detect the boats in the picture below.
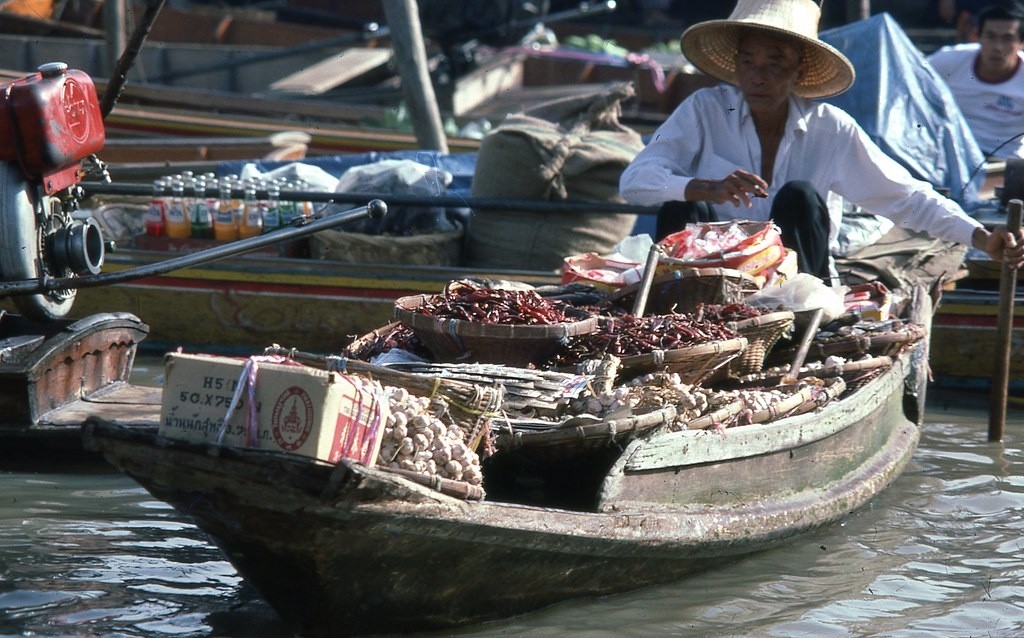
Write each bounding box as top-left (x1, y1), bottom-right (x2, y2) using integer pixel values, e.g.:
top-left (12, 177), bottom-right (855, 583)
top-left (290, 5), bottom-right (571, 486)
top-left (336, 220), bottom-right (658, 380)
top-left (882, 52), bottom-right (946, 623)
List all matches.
top-left (2, 0), bottom-right (1024, 638)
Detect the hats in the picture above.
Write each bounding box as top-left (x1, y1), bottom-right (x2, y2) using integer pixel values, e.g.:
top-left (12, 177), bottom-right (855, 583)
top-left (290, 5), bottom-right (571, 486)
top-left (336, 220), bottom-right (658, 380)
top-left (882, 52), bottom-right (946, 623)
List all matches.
top-left (680, 0), bottom-right (856, 101)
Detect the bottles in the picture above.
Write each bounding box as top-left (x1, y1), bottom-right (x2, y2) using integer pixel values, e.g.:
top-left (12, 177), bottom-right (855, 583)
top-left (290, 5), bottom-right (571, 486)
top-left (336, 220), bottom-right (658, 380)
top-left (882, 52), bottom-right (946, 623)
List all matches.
top-left (147, 171), bottom-right (314, 251)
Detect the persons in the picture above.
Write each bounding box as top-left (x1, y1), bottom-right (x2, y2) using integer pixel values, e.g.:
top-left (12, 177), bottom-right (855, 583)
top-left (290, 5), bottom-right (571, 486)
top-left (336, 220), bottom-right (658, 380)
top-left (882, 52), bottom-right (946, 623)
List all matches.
top-left (619, 0), bottom-right (1024, 285)
top-left (927, 0), bottom-right (1024, 159)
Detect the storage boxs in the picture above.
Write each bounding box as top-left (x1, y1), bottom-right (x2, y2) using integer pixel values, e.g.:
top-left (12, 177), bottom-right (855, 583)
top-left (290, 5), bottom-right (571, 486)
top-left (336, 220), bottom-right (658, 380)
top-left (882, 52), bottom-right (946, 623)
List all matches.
top-left (157, 351), bottom-right (387, 468)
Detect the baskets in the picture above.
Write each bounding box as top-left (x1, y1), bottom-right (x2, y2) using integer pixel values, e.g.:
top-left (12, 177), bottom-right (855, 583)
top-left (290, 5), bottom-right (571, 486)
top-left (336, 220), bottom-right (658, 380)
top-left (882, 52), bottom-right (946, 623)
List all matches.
top-left (266, 267), bottom-right (926, 501)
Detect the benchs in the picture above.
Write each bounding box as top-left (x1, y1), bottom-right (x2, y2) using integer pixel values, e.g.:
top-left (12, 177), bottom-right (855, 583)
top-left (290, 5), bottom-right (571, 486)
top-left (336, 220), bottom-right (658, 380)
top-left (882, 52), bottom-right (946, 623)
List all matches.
top-left (268, 46), bottom-right (394, 97)
